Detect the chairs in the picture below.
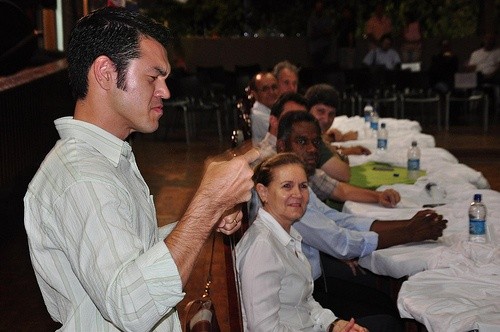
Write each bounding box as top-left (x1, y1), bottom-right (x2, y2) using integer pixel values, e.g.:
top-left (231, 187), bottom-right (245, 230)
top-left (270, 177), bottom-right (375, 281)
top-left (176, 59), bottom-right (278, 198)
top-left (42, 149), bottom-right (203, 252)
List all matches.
top-left (163, 60), bottom-right (499, 148)
top-left (223, 203), bottom-right (249, 332)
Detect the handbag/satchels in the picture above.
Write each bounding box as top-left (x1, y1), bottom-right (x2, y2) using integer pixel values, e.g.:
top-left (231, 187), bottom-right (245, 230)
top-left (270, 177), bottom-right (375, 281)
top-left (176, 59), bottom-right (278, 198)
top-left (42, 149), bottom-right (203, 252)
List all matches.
top-left (362, 62), bottom-right (381, 88)
top-left (182, 294), bottom-right (219, 332)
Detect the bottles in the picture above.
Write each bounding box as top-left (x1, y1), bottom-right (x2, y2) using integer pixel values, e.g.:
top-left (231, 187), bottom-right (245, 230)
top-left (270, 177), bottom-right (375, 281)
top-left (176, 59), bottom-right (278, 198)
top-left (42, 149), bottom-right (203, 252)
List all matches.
top-left (469, 193), bottom-right (487, 244)
top-left (364, 101), bottom-right (379, 139)
top-left (407, 141), bottom-right (420, 182)
top-left (377, 123), bottom-right (388, 154)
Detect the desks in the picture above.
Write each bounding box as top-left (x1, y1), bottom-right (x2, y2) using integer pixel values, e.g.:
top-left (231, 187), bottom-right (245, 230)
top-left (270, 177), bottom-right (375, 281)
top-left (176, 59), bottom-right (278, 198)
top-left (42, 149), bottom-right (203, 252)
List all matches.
top-left (330, 114), bottom-right (500, 332)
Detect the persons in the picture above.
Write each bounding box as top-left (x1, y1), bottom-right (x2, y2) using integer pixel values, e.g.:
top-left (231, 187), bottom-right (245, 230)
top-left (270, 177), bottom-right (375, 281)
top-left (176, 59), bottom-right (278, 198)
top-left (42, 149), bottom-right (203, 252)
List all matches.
top-left (225, 0), bottom-right (500, 332)
top-left (24, 5), bottom-right (261, 332)
